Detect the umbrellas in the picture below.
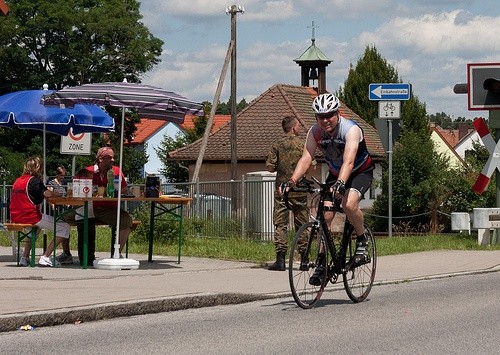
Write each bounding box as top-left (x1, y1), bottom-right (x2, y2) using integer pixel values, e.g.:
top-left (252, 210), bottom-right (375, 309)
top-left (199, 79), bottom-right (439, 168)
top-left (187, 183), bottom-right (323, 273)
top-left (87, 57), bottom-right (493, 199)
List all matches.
top-left (0, 83), bottom-right (115, 214)
top-left (40, 78), bottom-right (204, 270)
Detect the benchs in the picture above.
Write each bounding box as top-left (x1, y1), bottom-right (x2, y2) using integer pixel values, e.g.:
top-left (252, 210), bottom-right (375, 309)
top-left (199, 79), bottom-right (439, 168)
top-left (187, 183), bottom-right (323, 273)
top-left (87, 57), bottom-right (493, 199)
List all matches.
top-left (3, 220), bottom-right (141, 268)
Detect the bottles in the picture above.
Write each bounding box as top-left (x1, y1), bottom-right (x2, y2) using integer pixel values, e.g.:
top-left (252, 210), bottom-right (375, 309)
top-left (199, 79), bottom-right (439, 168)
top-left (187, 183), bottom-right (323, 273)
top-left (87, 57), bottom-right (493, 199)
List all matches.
top-left (113, 176), bottom-right (119, 198)
top-left (146, 176), bottom-right (160, 198)
top-left (106, 169), bottom-right (114, 198)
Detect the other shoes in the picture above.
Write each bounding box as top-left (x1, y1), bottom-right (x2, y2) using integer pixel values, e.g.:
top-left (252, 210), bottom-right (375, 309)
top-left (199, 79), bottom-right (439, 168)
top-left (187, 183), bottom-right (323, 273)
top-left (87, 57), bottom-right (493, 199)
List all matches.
top-left (39, 255), bottom-right (61, 267)
top-left (55, 253), bottom-right (74, 264)
top-left (309, 264), bottom-right (325, 286)
top-left (20, 257), bottom-right (30, 266)
top-left (351, 236), bottom-right (371, 265)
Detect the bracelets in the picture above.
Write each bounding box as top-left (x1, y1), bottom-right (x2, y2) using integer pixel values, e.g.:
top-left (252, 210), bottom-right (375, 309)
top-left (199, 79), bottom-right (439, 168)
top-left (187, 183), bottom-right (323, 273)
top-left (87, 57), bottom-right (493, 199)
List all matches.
top-left (129, 187), bottom-right (134, 194)
top-left (289, 178), bottom-right (297, 186)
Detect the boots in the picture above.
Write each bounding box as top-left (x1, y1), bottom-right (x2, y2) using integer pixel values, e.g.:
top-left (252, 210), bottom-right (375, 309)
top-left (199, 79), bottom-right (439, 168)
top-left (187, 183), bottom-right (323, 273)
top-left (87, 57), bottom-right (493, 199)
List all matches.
top-left (300, 253), bottom-right (309, 271)
top-left (268, 253), bottom-right (286, 271)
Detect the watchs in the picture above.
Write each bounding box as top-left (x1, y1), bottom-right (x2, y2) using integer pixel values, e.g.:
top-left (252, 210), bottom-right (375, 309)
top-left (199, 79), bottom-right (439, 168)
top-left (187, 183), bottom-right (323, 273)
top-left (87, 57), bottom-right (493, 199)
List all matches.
top-left (336, 179), bottom-right (346, 185)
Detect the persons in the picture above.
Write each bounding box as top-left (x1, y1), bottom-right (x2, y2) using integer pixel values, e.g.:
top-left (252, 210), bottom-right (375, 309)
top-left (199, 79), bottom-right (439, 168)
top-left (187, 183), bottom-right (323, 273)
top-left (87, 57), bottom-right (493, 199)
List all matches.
top-left (42, 166), bottom-right (67, 216)
top-left (10, 156), bottom-right (71, 267)
top-left (265, 115), bottom-right (315, 271)
top-left (55, 147), bottom-right (144, 264)
top-left (279, 93), bottom-right (376, 286)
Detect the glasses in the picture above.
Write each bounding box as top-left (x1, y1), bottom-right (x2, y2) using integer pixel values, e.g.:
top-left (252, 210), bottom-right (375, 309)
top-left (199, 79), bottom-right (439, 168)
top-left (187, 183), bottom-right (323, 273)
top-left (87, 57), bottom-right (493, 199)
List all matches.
top-left (317, 112), bottom-right (335, 120)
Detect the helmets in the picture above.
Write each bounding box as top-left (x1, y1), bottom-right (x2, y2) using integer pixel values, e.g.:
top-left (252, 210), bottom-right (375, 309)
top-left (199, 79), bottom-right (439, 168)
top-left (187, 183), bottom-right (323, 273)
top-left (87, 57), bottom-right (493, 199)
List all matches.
top-left (312, 93), bottom-right (340, 114)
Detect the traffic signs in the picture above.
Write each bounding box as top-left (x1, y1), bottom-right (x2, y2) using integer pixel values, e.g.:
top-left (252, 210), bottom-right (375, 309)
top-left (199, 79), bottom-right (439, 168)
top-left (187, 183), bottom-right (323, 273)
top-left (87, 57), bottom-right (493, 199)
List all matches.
top-left (369, 83), bottom-right (410, 100)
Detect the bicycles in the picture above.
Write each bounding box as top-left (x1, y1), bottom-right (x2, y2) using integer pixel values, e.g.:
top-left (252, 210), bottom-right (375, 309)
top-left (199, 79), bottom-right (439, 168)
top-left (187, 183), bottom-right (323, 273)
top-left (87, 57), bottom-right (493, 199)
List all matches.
top-left (282, 175), bottom-right (378, 309)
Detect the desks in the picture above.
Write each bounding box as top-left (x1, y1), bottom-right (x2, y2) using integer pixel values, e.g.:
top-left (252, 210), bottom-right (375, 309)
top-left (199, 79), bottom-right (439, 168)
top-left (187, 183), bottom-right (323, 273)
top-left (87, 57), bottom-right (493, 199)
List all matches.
top-left (48, 196), bottom-right (192, 269)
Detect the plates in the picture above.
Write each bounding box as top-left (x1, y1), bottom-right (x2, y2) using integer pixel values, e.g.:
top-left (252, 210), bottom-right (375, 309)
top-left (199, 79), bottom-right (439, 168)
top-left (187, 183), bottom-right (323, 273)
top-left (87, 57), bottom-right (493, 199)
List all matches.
top-left (122, 195), bottom-right (136, 198)
top-left (161, 194), bottom-right (181, 198)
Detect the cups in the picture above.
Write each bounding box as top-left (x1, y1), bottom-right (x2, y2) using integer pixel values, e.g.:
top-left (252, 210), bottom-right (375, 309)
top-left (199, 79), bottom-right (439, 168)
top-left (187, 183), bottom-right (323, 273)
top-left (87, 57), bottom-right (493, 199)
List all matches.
top-left (67, 183), bottom-right (73, 197)
top-left (92, 184), bottom-right (98, 198)
top-left (98, 187), bottom-right (104, 198)
